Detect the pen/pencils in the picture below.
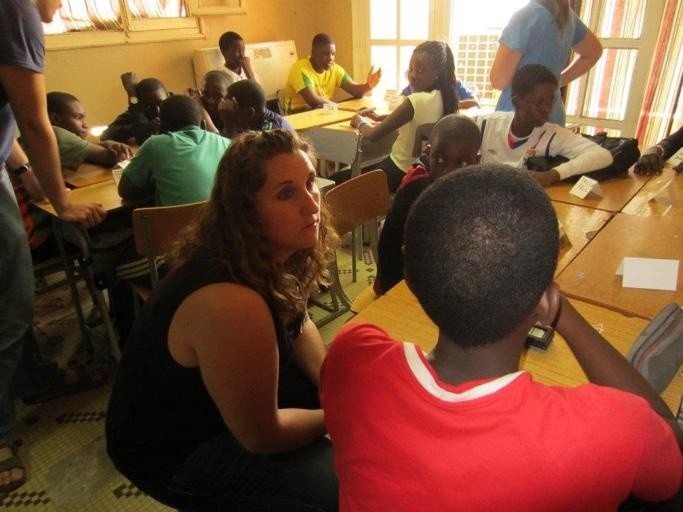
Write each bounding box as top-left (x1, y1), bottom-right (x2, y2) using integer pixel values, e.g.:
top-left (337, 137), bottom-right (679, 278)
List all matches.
top-left (357, 108), bottom-right (377, 114)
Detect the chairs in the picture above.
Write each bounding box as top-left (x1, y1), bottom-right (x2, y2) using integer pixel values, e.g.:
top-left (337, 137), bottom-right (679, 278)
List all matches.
top-left (275, 88), bottom-right (287, 115)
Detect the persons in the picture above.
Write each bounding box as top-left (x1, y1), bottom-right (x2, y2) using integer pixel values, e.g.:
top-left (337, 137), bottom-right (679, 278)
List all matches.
top-left (630, 123), bottom-right (683, 177)
top-left (100, 28), bottom-right (294, 358)
top-left (349, 1), bottom-right (612, 298)
top-left (107, 130), bottom-right (341, 511)
top-left (318, 163), bottom-right (683, 512)
top-left (281, 34), bottom-right (382, 176)
top-left (2, 0), bottom-right (118, 491)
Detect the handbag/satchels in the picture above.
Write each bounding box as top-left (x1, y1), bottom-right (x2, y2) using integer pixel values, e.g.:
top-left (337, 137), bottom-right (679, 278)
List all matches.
top-left (525, 131), bottom-right (640, 181)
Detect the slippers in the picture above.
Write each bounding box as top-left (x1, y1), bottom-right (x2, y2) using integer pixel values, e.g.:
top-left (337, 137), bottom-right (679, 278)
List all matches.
top-left (21, 361), bottom-right (110, 403)
top-left (0, 442), bottom-right (27, 492)
